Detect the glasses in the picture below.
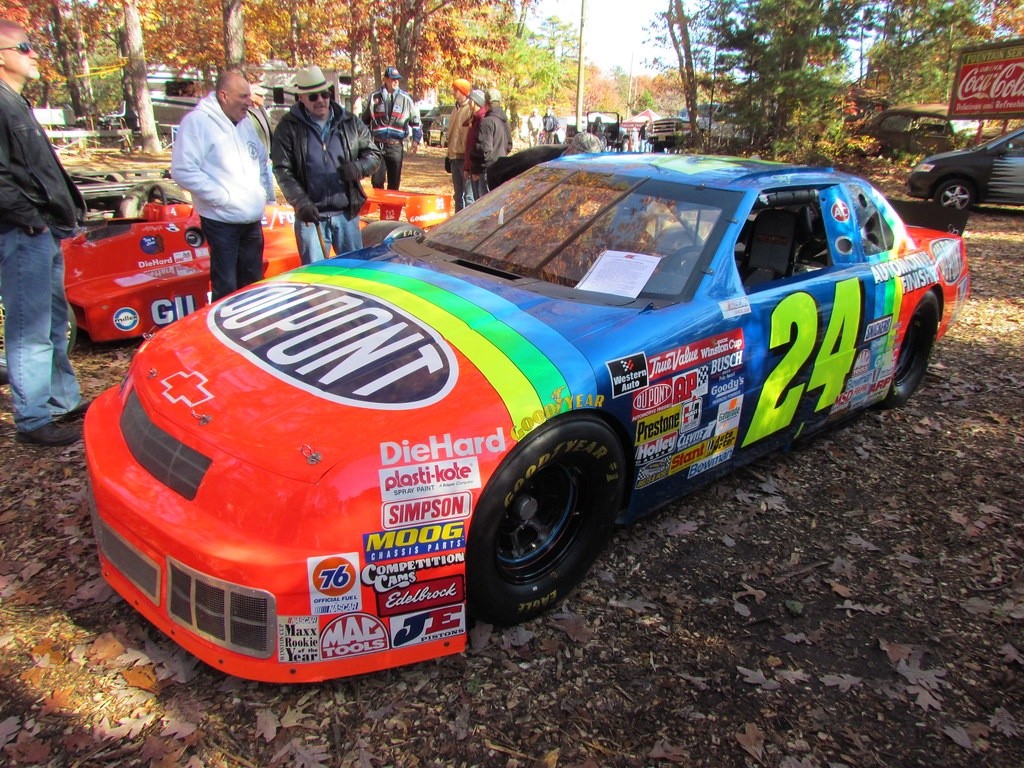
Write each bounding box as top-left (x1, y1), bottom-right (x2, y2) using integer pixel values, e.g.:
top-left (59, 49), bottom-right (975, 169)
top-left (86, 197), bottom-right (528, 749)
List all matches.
top-left (307, 92), bottom-right (330, 102)
top-left (0, 42), bottom-right (31, 52)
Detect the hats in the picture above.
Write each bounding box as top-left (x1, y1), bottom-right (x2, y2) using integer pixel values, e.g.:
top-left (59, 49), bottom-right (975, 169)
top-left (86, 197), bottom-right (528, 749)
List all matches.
top-left (385, 67), bottom-right (403, 80)
top-left (566, 133), bottom-right (602, 156)
top-left (453, 78), bottom-right (471, 96)
top-left (486, 89), bottom-right (501, 103)
top-left (284, 65), bottom-right (334, 94)
top-left (470, 89), bottom-right (486, 108)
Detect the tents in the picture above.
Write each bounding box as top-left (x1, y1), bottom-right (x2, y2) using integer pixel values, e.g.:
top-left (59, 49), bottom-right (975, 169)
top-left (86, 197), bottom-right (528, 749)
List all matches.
top-left (620, 109), bottom-right (662, 128)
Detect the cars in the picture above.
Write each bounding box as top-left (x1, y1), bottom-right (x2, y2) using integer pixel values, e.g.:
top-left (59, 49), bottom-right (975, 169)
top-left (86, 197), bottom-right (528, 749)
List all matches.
top-left (429, 113), bottom-right (453, 146)
top-left (909, 123), bottom-right (1024, 211)
top-left (853, 104), bottom-right (980, 153)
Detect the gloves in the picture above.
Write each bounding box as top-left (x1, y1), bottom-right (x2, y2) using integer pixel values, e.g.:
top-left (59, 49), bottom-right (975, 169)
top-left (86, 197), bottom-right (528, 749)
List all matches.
top-left (336, 155), bottom-right (358, 182)
top-left (301, 206), bottom-right (321, 226)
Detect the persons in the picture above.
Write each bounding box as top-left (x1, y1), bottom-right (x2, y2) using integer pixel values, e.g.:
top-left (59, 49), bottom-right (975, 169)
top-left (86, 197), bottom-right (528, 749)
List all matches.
top-left (0, 21), bottom-right (91, 445)
top-left (362, 65), bottom-right (424, 190)
top-left (527, 108), bottom-right (653, 154)
top-left (272, 66), bottom-right (379, 265)
top-left (449, 79), bottom-right (474, 213)
top-left (463, 89), bottom-right (489, 201)
top-left (476, 87), bottom-right (512, 197)
top-left (172, 72), bottom-right (274, 306)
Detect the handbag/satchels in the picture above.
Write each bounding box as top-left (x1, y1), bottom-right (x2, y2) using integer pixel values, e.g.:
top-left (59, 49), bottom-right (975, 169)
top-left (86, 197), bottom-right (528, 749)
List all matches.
top-left (445, 156), bottom-right (451, 173)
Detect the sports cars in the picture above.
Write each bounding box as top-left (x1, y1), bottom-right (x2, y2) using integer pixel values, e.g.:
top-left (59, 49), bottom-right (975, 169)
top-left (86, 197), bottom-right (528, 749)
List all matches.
top-left (0, 192), bottom-right (465, 377)
top-left (78, 152), bottom-right (964, 686)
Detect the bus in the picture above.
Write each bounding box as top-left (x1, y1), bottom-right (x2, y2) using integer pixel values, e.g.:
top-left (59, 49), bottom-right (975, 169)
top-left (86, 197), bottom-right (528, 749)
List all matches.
top-left (144, 67), bottom-right (364, 137)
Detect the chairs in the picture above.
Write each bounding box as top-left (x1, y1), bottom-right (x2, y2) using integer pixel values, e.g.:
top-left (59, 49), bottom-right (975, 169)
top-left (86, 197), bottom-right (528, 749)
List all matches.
top-left (105, 101), bottom-right (129, 131)
top-left (739, 209), bottom-right (804, 296)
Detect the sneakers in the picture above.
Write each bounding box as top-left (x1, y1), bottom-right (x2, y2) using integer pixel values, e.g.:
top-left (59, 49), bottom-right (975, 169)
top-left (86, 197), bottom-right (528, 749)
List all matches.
top-left (56, 398), bottom-right (91, 423)
top-left (15, 422), bottom-right (79, 446)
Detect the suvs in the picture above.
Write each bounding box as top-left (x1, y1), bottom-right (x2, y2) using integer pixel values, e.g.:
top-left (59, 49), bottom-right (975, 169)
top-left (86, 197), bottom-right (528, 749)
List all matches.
top-left (645, 101), bottom-right (754, 149)
top-left (420, 106), bottom-right (454, 139)
top-left (567, 110), bottom-right (630, 152)
